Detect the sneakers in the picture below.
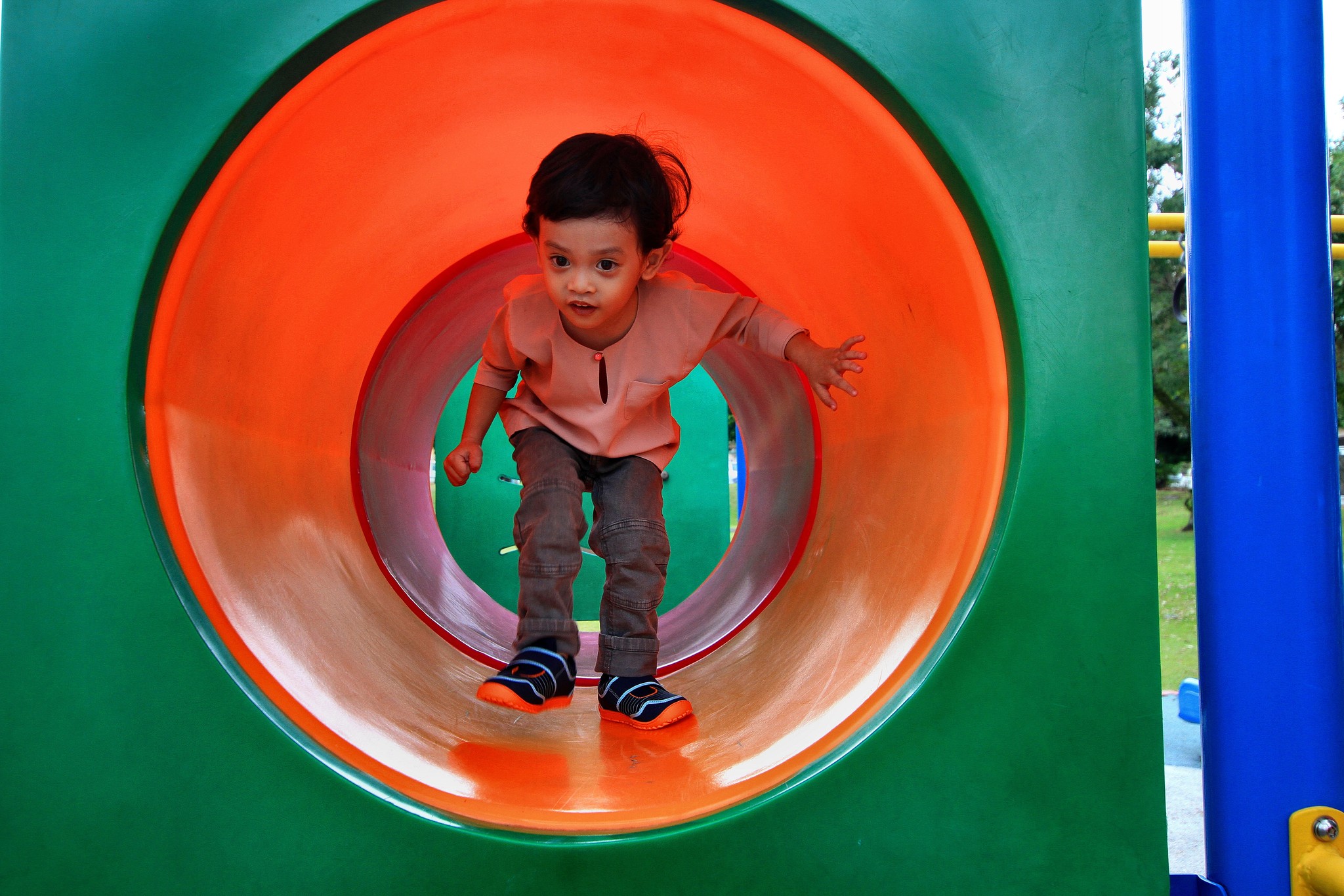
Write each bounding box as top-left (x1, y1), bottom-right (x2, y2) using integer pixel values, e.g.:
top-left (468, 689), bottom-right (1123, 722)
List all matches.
top-left (476, 647), bottom-right (577, 714)
top-left (598, 673), bottom-right (692, 730)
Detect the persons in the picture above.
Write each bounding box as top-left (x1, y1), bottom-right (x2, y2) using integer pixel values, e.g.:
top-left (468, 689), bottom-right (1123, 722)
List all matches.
top-left (439, 132), bottom-right (864, 730)
